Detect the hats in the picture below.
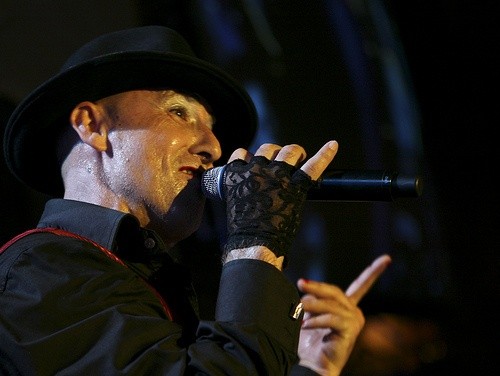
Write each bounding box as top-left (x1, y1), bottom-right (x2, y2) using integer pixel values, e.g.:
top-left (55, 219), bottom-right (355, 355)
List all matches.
top-left (3, 27), bottom-right (258, 199)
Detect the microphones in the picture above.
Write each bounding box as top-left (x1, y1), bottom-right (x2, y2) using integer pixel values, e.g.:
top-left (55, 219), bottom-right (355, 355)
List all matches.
top-left (202, 165), bottom-right (425, 205)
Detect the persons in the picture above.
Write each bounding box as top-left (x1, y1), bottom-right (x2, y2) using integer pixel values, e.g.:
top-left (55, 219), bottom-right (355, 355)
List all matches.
top-left (0, 28), bottom-right (393, 376)
